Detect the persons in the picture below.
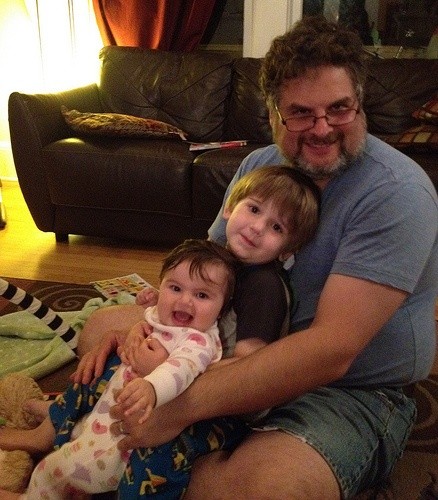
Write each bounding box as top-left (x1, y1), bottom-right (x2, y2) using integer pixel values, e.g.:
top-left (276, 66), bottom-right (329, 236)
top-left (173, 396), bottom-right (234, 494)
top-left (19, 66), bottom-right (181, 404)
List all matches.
top-left (0, 21), bottom-right (438, 500)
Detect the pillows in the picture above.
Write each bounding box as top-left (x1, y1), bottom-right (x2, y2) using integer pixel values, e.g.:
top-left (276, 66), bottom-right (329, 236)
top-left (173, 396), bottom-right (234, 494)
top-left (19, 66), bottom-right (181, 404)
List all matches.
top-left (60, 105), bottom-right (189, 141)
top-left (396, 93), bottom-right (438, 149)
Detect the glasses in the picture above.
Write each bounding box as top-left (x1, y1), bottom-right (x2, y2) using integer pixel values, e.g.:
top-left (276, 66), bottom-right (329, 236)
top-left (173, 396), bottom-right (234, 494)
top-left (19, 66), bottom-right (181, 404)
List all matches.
top-left (275, 102), bottom-right (361, 132)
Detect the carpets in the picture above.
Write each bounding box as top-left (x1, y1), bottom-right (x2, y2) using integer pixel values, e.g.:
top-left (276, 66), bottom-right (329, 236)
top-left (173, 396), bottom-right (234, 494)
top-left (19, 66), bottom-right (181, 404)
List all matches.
top-left (0, 275), bottom-right (438, 500)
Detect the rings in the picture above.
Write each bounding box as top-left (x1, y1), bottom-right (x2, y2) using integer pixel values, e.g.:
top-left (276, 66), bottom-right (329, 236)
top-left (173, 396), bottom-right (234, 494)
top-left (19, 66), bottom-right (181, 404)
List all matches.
top-left (118, 420), bottom-right (124, 433)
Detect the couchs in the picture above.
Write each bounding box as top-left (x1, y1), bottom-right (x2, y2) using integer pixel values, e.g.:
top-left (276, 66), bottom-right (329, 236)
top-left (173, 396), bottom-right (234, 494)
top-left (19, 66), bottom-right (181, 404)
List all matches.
top-left (8, 45), bottom-right (438, 245)
